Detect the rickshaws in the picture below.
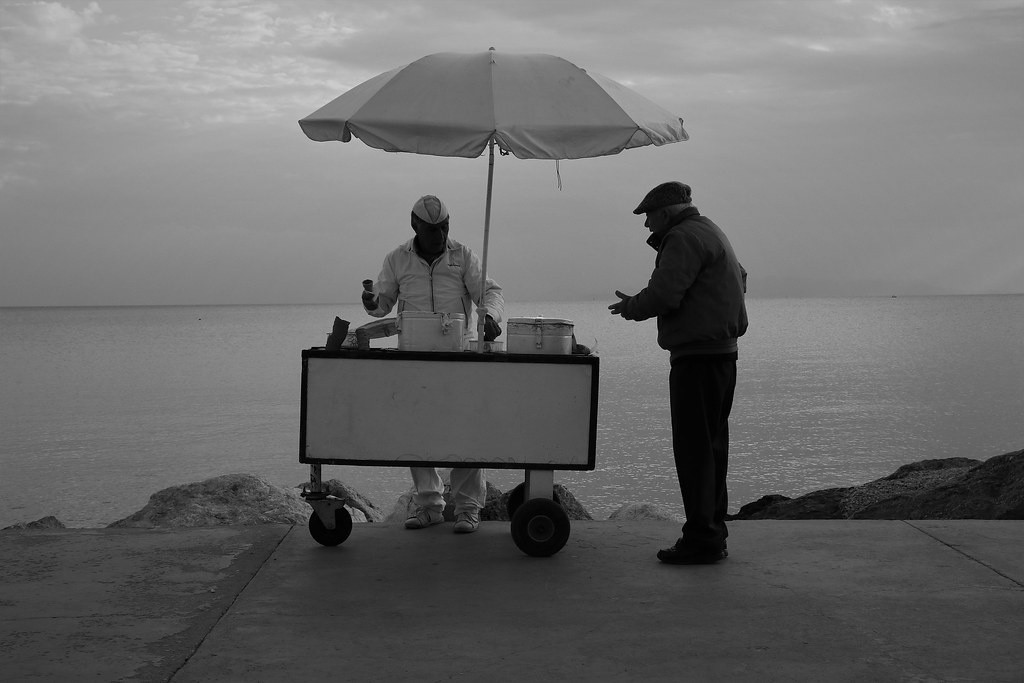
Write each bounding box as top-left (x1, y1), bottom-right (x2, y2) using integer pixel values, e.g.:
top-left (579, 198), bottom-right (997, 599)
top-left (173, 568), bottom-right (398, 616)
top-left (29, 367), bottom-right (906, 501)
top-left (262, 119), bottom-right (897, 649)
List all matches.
top-left (299, 346), bottom-right (600, 558)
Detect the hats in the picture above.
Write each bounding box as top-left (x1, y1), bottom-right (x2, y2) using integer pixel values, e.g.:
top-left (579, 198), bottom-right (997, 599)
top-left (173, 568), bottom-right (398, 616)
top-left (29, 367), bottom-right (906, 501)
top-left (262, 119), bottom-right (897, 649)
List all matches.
top-left (412, 194), bottom-right (449, 224)
top-left (633, 181), bottom-right (692, 215)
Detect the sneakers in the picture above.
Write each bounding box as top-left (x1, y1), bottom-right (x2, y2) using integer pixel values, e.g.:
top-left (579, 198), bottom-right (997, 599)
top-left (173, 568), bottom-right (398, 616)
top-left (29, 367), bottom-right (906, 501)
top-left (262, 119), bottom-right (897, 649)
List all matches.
top-left (405, 507), bottom-right (444, 529)
top-left (453, 512), bottom-right (479, 532)
top-left (657, 538), bottom-right (728, 564)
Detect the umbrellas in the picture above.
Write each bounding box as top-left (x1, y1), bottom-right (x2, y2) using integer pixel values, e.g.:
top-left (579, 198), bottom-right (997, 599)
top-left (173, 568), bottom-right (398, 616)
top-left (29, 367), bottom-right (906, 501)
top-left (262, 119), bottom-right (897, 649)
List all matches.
top-left (299, 45), bottom-right (688, 353)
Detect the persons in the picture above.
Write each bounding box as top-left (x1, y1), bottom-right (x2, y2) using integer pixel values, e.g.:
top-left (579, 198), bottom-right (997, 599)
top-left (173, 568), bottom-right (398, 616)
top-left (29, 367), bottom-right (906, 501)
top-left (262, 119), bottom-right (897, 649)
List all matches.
top-left (606, 181), bottom-right (748, 563)
top-left (359, 194), bottom-right (506, 535)
